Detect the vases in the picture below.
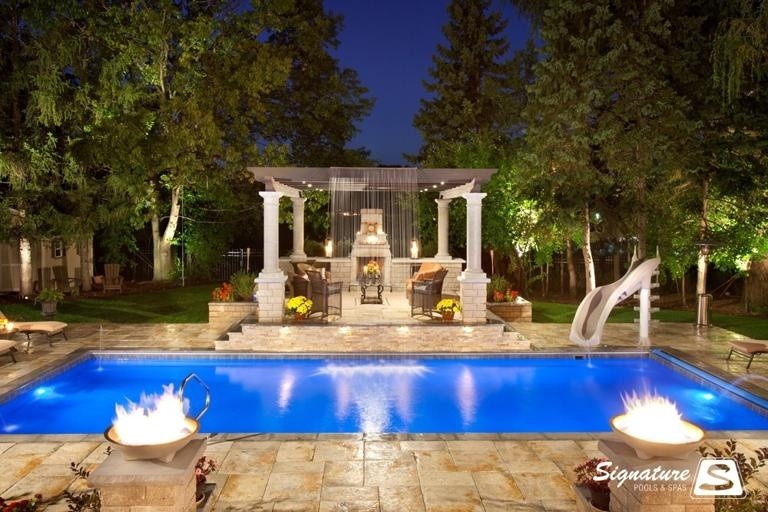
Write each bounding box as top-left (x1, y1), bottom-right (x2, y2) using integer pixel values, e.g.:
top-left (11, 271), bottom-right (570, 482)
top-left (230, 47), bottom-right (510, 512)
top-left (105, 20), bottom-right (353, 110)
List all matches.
top-left (194, 475), bottom-right (206, 502)
top-left (443, 310), bottom-right (454, 320)
top-left (589, 481), bottom-right (610, 508)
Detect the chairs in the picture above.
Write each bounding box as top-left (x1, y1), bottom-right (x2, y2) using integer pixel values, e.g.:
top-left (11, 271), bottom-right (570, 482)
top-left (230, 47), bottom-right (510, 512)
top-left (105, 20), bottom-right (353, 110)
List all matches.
top-left (30, 264), bottom-right (123, 302)
top-left (280, 259), bottom-right (343, 320)
top-left (0, 309), bottom-right (68, 363)
top-left (405, 260), bottom-right (448, 318)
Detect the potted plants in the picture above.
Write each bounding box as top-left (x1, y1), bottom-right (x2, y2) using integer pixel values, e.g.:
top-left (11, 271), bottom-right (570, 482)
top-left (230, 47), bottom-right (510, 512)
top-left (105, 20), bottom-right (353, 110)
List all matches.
top-left (33, 290), bottom-right (63, 317)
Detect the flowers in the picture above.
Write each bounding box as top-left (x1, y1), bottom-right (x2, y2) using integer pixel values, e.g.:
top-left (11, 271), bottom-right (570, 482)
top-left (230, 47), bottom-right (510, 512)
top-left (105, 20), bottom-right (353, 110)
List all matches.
top-left (193, 457), bottom-right (216, 482)
top-left (434, 297), bottom-right (461, 313)
top-left (211, 282), bottom-right (235, 303)
top-left (494, 288), bottom-right (518, 302)
top-left (573, 456), bottom-right (612, 488)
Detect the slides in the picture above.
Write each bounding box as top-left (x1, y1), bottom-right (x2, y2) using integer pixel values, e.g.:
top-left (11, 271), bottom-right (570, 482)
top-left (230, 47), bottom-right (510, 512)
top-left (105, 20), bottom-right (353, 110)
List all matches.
top-left (569, 255), bottom-right (661, 349)
